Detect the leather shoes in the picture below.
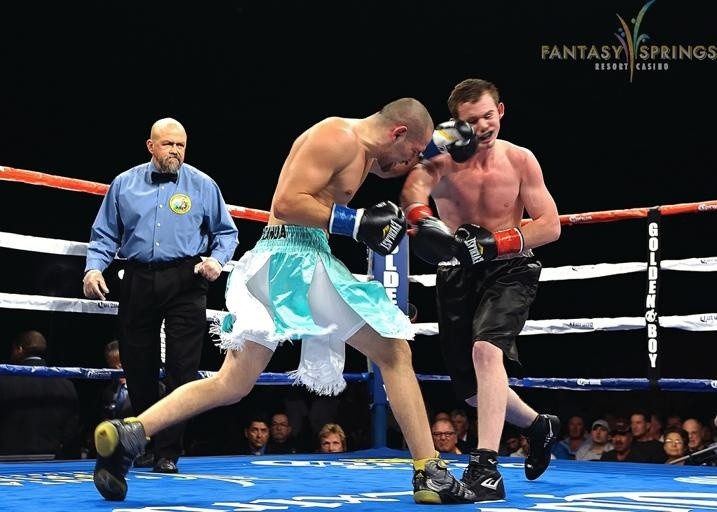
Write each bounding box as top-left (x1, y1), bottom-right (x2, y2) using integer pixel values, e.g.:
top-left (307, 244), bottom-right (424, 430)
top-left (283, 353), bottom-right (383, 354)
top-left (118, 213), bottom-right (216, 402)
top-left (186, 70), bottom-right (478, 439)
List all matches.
top-left (133, 452), bottom-right (156, 469)
top-left (157, 457), bottom-right (177, 473)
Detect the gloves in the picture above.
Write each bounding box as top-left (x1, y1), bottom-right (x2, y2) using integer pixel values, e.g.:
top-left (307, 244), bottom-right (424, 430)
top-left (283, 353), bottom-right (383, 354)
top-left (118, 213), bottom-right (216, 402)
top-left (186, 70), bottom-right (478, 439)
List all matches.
top-left (405, 203), bottom-right (456, 264)
top-left (419, 118), bottom-right (479, 163)
top-left (453, 222), bottom-right (523, 266)
top-left (327, 200), bottom-right (407, 257)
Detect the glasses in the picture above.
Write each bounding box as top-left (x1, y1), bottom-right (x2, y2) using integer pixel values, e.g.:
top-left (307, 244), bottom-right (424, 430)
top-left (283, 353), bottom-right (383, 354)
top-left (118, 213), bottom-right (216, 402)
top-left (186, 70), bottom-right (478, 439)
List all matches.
top-left (665, 438), bottom-right (682, 445)
top-left (433, 431), bottom-right (456, 438)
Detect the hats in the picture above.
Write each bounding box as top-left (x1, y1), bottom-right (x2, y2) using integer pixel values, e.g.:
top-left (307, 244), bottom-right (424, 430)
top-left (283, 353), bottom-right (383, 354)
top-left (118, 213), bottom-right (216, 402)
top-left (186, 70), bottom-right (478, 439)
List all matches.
top-left (608, 418), bottom-right (632, 434)
top-left (592, 419), bottom-right (608, 429)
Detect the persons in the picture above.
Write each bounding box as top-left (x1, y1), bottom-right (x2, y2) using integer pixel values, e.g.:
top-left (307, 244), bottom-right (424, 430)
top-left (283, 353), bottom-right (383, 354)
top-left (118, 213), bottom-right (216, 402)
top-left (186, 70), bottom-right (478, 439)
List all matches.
top-left (86, 94), bottom-right (479, 503)
top-left (80, 114), bottom-right (239, 478)
top-left (2, 326), bottom-right (716, 470)
top-left (400, 76), bottom-right (563, 503)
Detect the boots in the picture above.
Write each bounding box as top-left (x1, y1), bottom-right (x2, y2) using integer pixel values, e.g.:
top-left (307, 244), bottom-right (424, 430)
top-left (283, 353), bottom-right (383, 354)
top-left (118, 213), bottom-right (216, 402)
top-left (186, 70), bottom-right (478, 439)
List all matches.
top-left (413, 449), bottom-right (476, 503)
top-left (459, 448), bottom-right (506, 500)
top-left (520, 414), bottom-right (561, 480)
top-left (93, 416), bottom-right (151, 500)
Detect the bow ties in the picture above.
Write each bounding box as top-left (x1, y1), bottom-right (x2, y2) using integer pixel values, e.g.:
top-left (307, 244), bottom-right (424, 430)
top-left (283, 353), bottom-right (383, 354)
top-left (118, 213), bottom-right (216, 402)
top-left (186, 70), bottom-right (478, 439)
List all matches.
top-left (151, 172), bottom-right (177, 185)
